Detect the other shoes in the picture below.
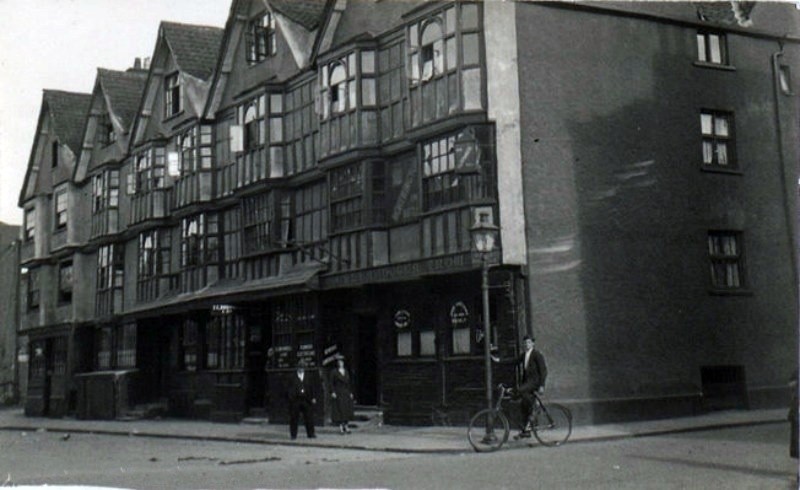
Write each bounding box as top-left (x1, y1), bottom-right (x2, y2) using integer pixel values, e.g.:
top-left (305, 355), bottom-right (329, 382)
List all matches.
top-left (345, 427), bottom-right (351, 433)
top-left (340, 429), bottom-right (345, 434)
top-left (519, 431), bottom-right (530, 437)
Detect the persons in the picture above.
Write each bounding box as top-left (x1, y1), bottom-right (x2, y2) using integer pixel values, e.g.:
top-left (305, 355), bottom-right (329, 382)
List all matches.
top-left (243, 346), bottom-right (276, 417)
top-left (785, 368), bottom-right (800, 482)
top-left (330, 355), bottom-right (357, 435)
top-left (289, 364), bottom-right (318, 440)
top-left (490, 334), bottom-right (548, 440)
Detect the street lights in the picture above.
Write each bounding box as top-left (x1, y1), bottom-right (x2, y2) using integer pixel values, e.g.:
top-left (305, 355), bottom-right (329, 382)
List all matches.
top-left (466, 211), bottom-right (502, 442)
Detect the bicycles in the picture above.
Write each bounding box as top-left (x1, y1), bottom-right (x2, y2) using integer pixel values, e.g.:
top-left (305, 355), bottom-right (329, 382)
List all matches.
top-left (466, 382), bottom-right (573, 453)
